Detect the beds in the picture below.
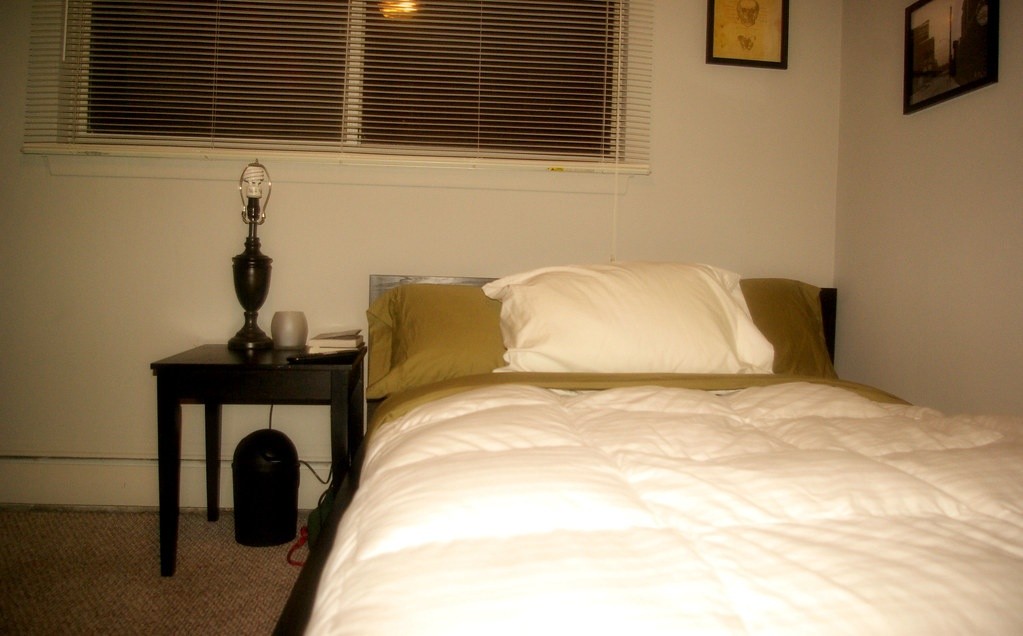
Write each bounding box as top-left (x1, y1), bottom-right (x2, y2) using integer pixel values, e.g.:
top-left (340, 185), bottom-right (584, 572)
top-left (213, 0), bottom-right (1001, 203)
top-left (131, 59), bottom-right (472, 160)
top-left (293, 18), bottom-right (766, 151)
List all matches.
top-left (301, 273), bottom-right (1023, 636)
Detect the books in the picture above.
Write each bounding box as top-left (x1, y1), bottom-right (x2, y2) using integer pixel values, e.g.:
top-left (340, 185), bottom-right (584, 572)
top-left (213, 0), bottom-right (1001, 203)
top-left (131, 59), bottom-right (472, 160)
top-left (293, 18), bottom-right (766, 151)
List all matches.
top-left (307, 328), bottom-right (365, 353)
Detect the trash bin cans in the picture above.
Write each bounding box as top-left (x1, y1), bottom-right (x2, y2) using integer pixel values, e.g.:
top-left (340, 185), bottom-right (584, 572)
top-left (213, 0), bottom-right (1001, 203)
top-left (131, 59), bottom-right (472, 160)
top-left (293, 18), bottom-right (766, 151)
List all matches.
top-left (231, 429), bottom-right (299, 547)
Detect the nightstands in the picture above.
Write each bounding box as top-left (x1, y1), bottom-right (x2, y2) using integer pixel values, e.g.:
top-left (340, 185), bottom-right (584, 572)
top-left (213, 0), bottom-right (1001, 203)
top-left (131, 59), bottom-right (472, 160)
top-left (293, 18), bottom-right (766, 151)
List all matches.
top-left (150, 343), bottom-right (368, 579)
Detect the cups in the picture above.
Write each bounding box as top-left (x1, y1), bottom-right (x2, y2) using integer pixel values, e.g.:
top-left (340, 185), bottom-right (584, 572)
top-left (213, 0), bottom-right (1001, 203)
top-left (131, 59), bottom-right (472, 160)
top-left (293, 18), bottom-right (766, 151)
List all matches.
top-left (270, 311), bottom-right (309, 351)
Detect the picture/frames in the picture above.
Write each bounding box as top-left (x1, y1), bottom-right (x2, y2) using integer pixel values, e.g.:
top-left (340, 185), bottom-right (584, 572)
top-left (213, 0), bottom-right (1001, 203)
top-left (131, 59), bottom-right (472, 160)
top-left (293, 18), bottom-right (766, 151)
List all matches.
top-left (903, 0), bottom-right (1000, 116)
top-left (706, 0), bottom-right (789, 70)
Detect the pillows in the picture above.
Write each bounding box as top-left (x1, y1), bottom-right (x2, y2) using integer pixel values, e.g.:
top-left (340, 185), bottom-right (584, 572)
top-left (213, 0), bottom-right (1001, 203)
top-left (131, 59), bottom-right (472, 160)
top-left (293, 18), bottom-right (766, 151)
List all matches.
top-left (740, 278), bottom-right (839, 377)
top-left (366, 281), bottom-right (508, 400)
top-left (480, 261), bottom-right (775, 375)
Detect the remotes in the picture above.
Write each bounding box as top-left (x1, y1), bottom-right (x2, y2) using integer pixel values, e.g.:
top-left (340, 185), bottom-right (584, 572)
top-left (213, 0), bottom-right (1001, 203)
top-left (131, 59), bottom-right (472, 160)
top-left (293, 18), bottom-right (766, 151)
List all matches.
top-left (286, 349), bottom-right (359, 364)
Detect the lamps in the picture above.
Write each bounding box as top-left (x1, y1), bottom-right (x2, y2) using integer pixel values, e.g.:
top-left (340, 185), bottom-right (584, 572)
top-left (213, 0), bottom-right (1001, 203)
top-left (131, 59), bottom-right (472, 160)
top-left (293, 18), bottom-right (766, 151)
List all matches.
top-left (228, 159), bottom-right (272, 349)
